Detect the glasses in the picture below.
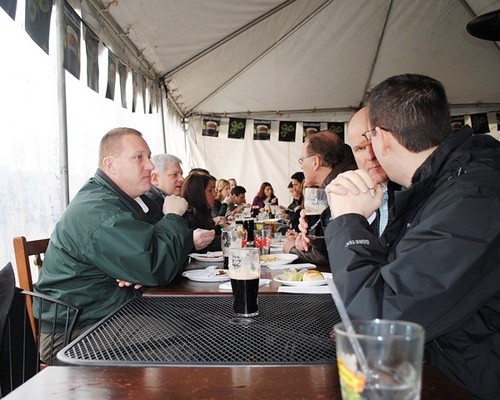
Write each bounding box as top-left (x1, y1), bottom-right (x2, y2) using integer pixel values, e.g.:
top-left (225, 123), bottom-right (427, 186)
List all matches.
top-left (298, 154), bottom-right (321, 164)
top-left (362, 126), bottom-right (390, 142)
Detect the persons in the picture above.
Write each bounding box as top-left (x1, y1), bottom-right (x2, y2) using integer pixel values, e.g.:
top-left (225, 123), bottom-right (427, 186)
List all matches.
top-left (252, 182), bottom-right (278, 209)
top-left (32, 127), bottom-right (251, 367)
top-left (276, 73), bottom-right (500, 400)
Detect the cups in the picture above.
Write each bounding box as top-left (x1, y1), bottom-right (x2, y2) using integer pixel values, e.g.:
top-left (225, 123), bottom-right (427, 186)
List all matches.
top-left (229, 248), bottom-right (260, 317)
top-left (221, 203), bottom-right (282, 269)
top-left (334, 320), bottom-right (425, 400)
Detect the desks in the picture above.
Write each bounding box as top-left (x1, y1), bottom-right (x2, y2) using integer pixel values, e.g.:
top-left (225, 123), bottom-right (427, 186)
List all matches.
top-left (0, 259), bottom-right (382, 400)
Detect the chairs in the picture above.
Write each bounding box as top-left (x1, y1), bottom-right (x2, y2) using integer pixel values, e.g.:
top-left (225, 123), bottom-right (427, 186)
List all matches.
top-left (0, 237), bottom-right (82, 399)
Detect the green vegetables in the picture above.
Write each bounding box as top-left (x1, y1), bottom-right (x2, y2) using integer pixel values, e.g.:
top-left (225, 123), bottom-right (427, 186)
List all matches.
top-left (276, 270), bottom-right (303, 281)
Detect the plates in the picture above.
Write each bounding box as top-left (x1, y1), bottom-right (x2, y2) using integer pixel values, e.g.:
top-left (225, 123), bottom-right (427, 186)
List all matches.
top-left (261, 254), bottom-right (298, 266)
top-left (190, 254), bottom-right (224, 262)
top-left (183, 269), bottom-right (229, 282)
top-left (274, 272), bottom-right (333, 285)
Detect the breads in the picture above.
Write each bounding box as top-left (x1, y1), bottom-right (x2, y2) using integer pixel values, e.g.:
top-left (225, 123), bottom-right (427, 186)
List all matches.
top-left (303, 270), bottom-right (325, 281)
